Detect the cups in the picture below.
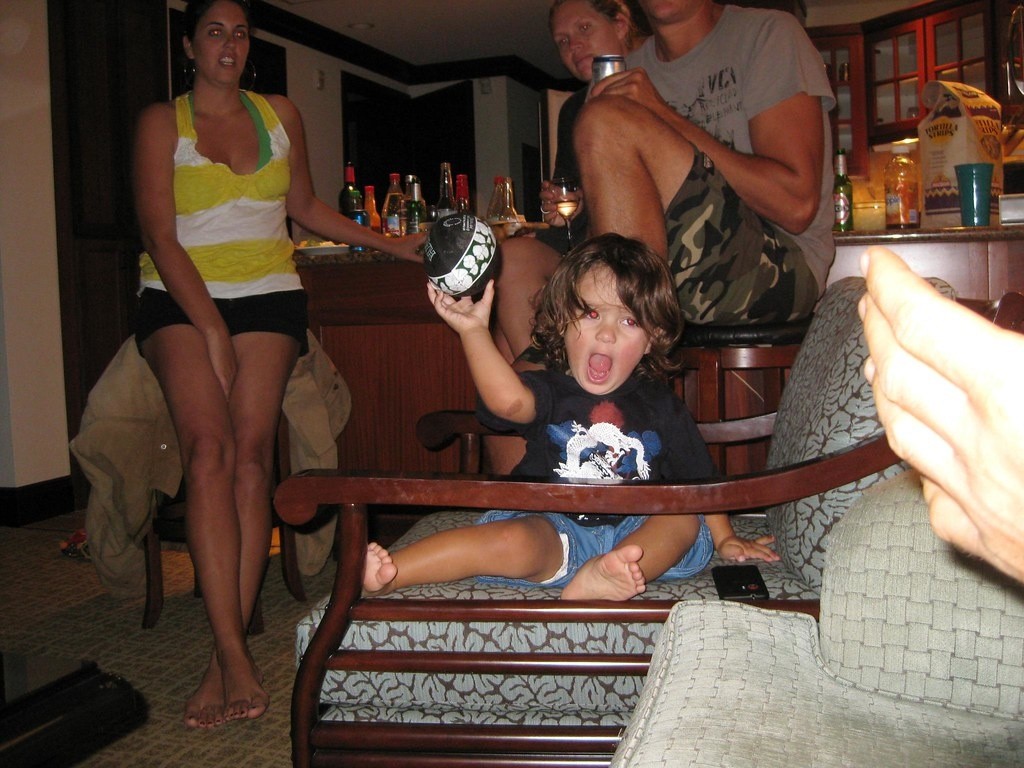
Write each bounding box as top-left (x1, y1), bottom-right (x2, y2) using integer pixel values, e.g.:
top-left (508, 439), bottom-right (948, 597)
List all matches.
top-left (953, 162), bottom-right (995, 227)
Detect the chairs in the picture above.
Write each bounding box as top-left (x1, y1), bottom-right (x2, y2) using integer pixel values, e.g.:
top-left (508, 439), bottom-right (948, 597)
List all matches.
top-left (271, 290), bottom-right (1024, 768)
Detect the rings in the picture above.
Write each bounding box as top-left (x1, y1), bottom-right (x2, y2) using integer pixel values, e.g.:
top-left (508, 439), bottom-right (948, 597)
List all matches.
top-left (541, 205), bottom-right (550, 214)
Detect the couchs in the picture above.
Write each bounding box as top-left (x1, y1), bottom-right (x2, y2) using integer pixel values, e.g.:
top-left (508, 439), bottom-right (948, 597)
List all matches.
top-left (608, 463), bottom-right (1024, 768)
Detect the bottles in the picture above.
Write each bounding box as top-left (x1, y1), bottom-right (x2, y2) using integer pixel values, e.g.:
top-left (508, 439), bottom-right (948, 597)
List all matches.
top-left (824, 61), bottom-right (849, 82)
top-left (338, 161), bottom-right (471, 250)
top-left (833, 148), bottom-right (854, 232)
top-left (486, 175), bottom-right (504, 221)
top-left (883, 145), bottom-right (920, 230)
top-left (499, 176), bottom-right (519, 222)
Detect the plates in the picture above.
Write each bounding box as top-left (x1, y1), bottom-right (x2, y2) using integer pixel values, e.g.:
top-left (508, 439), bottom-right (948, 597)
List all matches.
top-left (295, 245), bottom-right (350, 256)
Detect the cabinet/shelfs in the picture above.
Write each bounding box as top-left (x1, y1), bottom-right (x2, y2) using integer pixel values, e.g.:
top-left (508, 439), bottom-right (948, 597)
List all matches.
top-left (802, 0), bottom-right (1024, 178)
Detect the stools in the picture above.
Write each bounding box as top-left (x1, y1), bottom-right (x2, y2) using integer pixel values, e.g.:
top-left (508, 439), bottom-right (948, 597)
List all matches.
top-left (665, 312), bottom-right (815, 484)
top-left (67, 325), bottom-right (353, 637)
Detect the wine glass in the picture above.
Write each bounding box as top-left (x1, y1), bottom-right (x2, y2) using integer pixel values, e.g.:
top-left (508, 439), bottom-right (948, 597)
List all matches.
top-left (549, 176), bottom-right (579, 257)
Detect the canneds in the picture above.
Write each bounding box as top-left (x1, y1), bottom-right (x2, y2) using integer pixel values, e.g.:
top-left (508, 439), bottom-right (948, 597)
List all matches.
top-left (591, 54), bottom-right (626, 85)
top-left (349, 209), bottom-right (370, 251)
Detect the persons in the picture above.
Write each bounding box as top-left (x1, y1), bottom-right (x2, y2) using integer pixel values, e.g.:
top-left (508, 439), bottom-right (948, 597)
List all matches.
top-left (360, 229), bottom-right (780, 601)
top-left (539, 0), bottom-right (835, 326)
top-left (858, 246), bottom-right (1023, 587)
top-left (128, 0), bottom-right (428, 728)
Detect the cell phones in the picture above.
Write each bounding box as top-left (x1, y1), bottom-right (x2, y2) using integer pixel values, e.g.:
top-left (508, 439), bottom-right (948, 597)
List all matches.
top-left (711, 564), bottom-right (769, 601)
